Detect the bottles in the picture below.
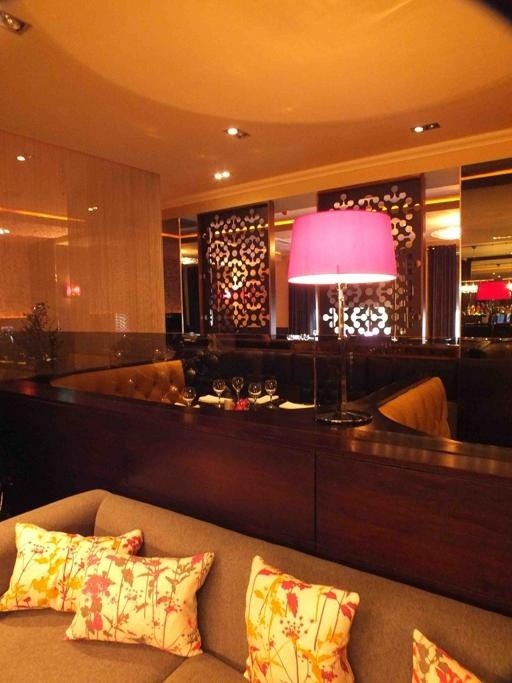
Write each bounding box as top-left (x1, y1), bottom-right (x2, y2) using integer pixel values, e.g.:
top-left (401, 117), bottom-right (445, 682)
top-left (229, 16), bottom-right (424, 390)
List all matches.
top-left (465, 301), bottom-right (512, 325)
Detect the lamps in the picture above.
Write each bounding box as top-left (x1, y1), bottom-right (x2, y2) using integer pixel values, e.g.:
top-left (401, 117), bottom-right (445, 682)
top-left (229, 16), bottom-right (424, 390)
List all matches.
top-left (285, 210), bottom-right (400, 428)
top-left (475, 279), bottom-right (510, 331)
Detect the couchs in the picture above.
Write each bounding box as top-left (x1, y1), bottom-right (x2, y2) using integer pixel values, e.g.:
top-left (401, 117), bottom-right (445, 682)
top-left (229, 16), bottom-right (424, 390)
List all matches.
top-left (0, 487), bottom-right (512, 683)
top-left (52, 355), bottom-right (512, 455)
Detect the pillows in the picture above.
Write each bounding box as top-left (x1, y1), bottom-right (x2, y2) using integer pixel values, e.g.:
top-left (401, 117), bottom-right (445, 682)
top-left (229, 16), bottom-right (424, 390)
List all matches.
top-left (2, 522), bottom-right (145, 615)
top-left (66, 547), bottom-right (215, 660)
top-left (412, 627), bottom-right (482, 682)
top-left (241, 554), bottom-right (360, 683)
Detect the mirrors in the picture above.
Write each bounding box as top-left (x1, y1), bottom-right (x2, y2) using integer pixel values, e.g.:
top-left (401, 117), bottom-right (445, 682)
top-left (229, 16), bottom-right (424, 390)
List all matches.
top-left (458, 176), bottom-right (512, 339)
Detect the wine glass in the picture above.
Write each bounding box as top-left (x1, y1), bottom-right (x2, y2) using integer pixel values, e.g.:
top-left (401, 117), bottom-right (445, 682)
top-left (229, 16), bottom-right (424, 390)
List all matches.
top-left (181, 376), bottom-right (280, 412)
